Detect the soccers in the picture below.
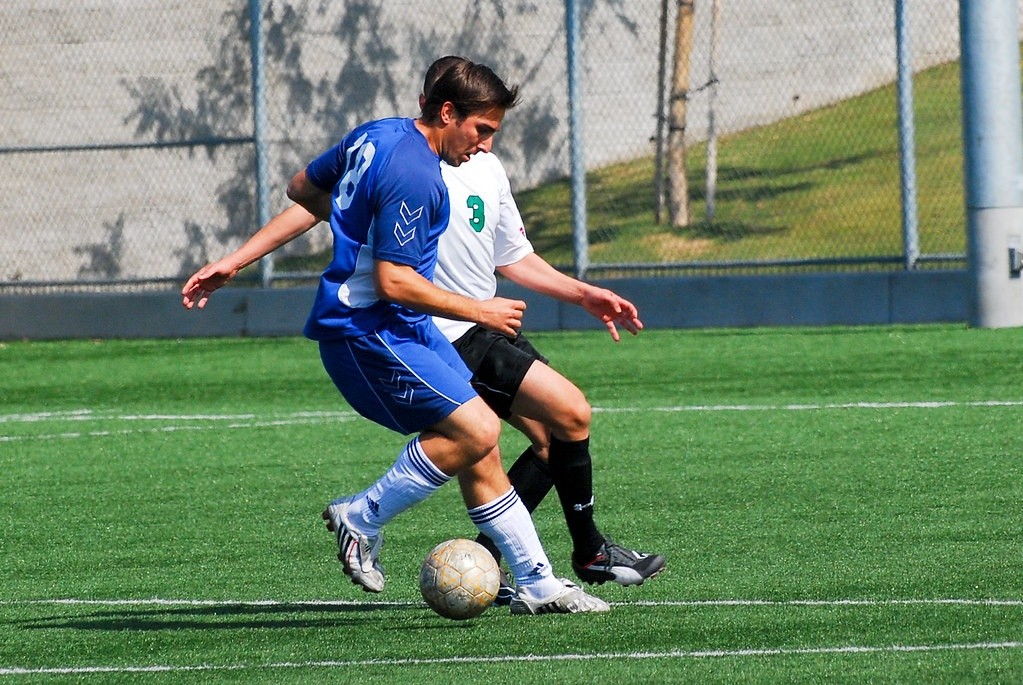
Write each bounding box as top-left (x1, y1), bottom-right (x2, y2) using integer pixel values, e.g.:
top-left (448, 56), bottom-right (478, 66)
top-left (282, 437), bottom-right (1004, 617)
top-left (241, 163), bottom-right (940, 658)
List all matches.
top-left (420, 536), bottom-right (501, 621)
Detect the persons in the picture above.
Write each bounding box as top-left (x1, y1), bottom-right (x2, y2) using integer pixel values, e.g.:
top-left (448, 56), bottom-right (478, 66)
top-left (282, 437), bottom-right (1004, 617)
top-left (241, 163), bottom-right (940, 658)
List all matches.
top-left (285, 61), bottom-right (614, 615)
top-left (180, 56), bottom-right (667, 607)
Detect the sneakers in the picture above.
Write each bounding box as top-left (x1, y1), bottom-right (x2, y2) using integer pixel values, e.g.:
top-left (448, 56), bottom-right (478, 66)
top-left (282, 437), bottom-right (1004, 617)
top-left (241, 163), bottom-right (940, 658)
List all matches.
top-left (490, 569), bottom-right (515, 605)
top-left (570, 538), bottom-right (664, 588)
top-left (322, 495), bottom-right (386, 594)
top-left (510, 578), bottom-right (611, 614)
